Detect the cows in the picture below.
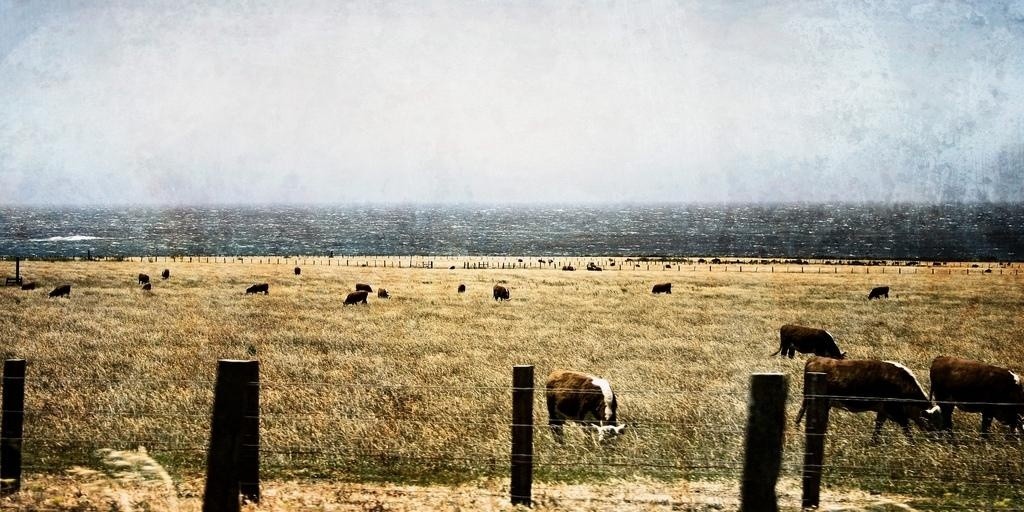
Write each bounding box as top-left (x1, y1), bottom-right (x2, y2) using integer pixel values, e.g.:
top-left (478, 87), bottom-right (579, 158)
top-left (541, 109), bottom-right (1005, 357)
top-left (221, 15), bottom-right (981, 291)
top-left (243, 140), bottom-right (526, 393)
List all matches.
top-left (929, 355), bottom-right (1024, 439)
top-left (378, 288), bottom-right (389, 299)
top-left (162, 269), bottom-right (169, 279)
top-left (21, 281), bottom-right (36, 291)
top-left (295, 267), bottom-right (300, 275)
top-left (770, 324), bottom-right (847, 360)
top-left (138, 273), bottom-right (151, 291)
top-left (795, 356), bottom-right (942, 446)
top-left (343, 283), bottom-right (373, 307)
top-left (246, 283), bottom-right (268, 295)
top-left (493, 284), bottom-right (510, 302)
top-left (458, 285), bottom-right (465, 293)
top-left (651, 282), bottom-right (673, 295)
top-left (868, 286), bottom-right (889, 300)
top-left (48, 284), bottom-right (71, 299)
top-left (546, 371), bottom-right (627, 449)
